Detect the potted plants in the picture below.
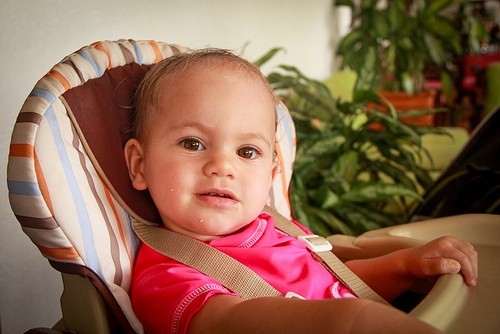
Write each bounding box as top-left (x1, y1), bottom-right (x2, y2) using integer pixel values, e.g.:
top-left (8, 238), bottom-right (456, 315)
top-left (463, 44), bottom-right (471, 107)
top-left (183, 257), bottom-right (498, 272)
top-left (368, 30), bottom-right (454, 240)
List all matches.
top-left (332, 0), bottom-right (500, 131)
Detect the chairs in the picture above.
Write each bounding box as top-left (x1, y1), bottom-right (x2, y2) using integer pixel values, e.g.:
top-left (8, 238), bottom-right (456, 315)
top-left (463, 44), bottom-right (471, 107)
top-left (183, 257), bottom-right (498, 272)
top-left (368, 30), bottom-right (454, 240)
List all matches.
top-left (7, 39), bottom-right (352, 334)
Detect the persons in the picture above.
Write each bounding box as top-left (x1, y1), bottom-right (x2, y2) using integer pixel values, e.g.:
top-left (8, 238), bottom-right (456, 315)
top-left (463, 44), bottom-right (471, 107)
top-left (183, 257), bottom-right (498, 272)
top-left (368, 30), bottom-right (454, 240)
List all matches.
top-left (123, 47), bottom-right (478, 334)
